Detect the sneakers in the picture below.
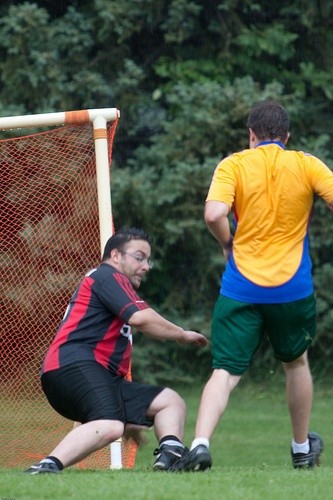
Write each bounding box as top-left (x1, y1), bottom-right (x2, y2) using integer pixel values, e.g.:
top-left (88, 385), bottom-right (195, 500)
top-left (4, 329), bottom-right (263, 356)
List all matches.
top-left (152, 444), bottom-right (189, 469)
top-left (22, 462), bottom-right (63, 476)
top-left (168, 444), bottom-right (213, 472)
top-left (290, 432), bottom-right (325, 469)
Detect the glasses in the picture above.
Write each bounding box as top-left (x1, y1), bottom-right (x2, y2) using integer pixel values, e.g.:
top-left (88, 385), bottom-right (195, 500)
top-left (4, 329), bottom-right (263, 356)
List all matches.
top-left (124, 251), bottom-right (154, 268)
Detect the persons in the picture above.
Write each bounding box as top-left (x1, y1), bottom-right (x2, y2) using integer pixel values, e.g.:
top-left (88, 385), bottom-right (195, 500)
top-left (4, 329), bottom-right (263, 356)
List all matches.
top-left (22, 226), bottom-right (209, 477)
top-left (166, 102), bottom-right (333, 473)
